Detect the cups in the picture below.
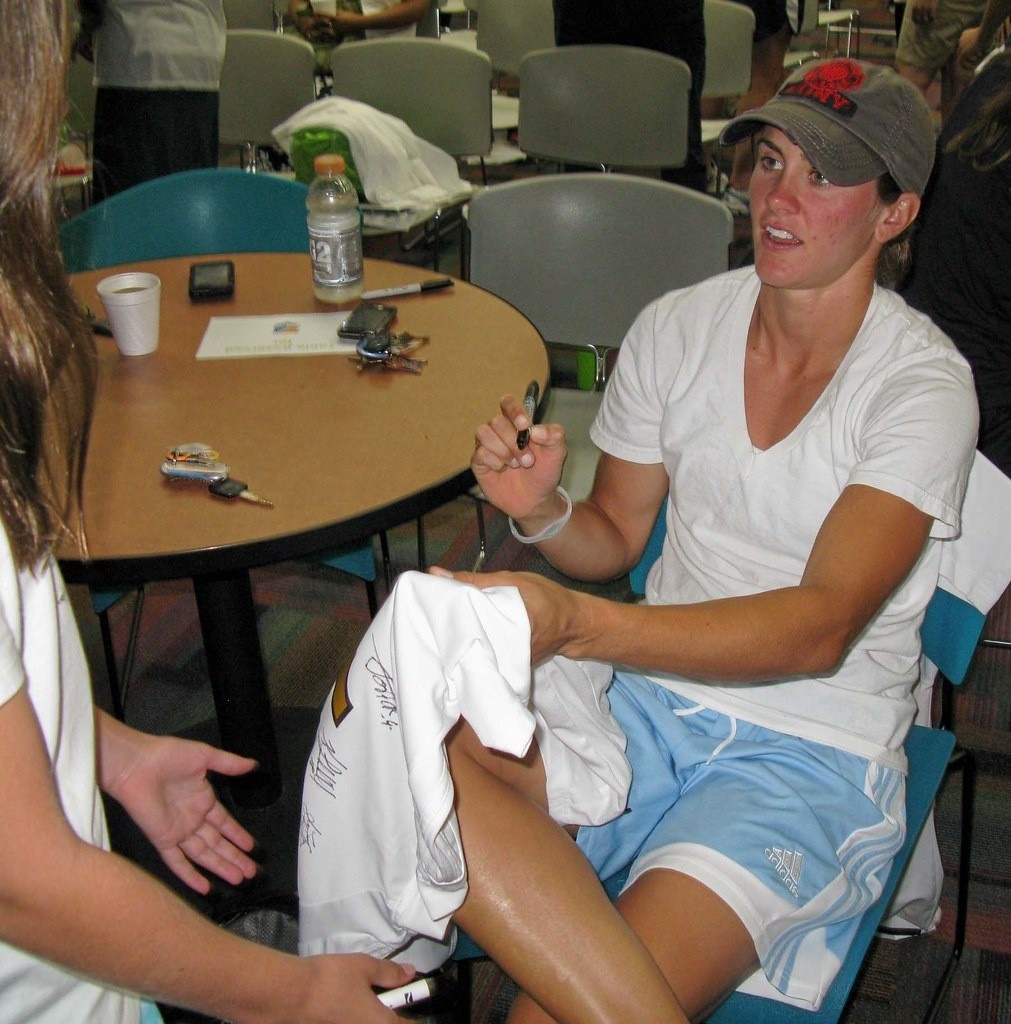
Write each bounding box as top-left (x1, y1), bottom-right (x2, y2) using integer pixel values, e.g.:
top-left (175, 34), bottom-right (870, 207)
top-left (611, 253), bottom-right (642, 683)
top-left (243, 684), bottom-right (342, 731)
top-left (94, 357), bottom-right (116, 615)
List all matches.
top-left (95, 270), bottom-right (161, 356)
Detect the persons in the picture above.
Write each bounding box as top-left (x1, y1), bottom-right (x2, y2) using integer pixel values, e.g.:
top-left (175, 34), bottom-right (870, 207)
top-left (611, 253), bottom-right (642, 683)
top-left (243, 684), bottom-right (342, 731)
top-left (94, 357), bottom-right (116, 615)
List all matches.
top-left (294, 0), bottom-right (435, 41)
top-left (885, 0), bottom-right (1011, 479)
top-left (548, 0), bottom-right (807, 218)
top-left (0, 0), bottom-right (441, 1024)
top-left (417, 66), bottom-right (979, 1024)
top-left (81, 0), bottom-right (229, 203)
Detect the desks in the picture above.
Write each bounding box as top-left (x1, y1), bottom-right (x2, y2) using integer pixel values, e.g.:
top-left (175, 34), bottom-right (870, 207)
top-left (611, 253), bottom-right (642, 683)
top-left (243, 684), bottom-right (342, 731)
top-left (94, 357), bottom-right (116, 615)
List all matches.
top-left (34, 252), bottom-right (552, 923)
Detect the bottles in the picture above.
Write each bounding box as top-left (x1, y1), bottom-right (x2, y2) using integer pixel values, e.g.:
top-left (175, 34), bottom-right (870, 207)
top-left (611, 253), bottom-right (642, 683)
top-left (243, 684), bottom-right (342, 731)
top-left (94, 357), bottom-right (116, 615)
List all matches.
top-left (305, 155), bottom-right (366, 304)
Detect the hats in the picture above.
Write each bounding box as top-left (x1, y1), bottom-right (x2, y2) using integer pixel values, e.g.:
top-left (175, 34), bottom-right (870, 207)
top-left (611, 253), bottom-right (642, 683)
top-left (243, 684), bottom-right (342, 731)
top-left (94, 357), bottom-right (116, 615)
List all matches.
top-left (719, 57), bottom-right (937, 197)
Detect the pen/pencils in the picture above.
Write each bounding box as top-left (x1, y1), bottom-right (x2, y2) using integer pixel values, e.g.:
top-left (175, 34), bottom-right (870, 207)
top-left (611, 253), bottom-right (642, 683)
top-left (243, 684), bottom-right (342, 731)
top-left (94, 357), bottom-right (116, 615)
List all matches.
top-left (363, 276), bottom-right (455, 301)
top-left (515, 379), bottom-right (540, 449)
top-left (369, 974), bottom-right (445, 1010)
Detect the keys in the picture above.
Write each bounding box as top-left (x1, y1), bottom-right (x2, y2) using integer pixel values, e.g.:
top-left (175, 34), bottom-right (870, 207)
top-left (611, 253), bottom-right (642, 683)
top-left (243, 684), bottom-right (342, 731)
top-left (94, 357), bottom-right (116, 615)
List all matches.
top-left (361, 328), bottom-right (429, 375)
top-left (208, 477), bottom-right (274, 507)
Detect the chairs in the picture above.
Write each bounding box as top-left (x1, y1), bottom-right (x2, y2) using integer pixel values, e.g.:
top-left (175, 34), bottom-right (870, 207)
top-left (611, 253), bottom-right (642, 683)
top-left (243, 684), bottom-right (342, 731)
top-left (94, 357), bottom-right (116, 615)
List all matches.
top-left (58, 166), bottom-right (378, 722)
top-left (474, 0), bottom-right (554, 79)
top-left (450, 447), bottom-right (1011, 1024)
top-left (331, 36), bottom-right (495, 280)
top-left (60, 53), bottom-right (97, 210)
top-left (700, 0), bottom-right (756, 200)
top-left (219, 26), bottom-right (316, 170)
top-left (223, 0), bottom-right (285, 34)
top-left (414, 171), bottom-right (733, 575)
top-left (516, 42), bottom-right (692, 180)
top-left (783, 51), bottom-right (820, 69)
top-left (817, 7), bottom-right (860, 61)
top-left (824, 0), bottom-right (897, 59)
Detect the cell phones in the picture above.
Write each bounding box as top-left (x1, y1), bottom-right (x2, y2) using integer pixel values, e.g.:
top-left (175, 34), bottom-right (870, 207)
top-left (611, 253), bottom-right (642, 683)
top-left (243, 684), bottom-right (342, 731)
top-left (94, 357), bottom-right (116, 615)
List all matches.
top-left (337, 300), bottom-right (398, 340)
top-left (189, 260), bottom-right (235, 300)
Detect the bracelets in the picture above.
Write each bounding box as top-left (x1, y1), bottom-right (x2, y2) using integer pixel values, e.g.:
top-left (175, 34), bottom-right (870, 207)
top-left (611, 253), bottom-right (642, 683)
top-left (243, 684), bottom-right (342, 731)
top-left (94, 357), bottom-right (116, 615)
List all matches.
top-left (507, 485), bottom-right (572, 544)
